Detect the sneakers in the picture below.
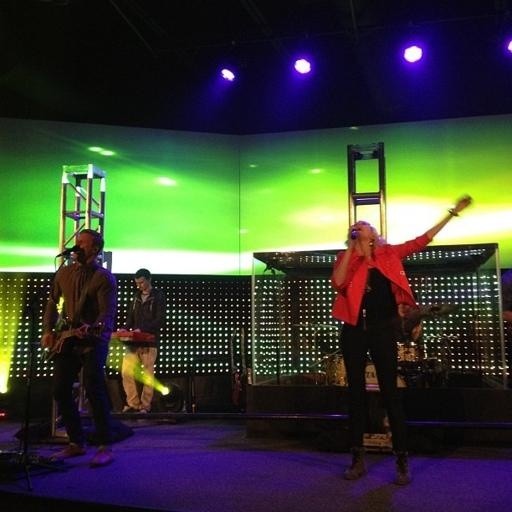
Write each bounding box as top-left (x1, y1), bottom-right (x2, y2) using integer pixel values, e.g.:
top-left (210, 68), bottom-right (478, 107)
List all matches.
top-left (52, 443), bottom-right (86, 458)
top-left (123, 406), bottom-right (147, 413)
top-left (90, 445), bottom-right (113, 467)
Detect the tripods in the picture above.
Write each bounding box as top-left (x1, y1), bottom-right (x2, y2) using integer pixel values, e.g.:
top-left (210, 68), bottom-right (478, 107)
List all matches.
top-left (0, 257), bottom-right (67, 491)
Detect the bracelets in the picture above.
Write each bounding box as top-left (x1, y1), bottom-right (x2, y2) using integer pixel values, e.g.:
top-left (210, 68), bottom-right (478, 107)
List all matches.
top-left (447, 209), bottom-right (459, 218)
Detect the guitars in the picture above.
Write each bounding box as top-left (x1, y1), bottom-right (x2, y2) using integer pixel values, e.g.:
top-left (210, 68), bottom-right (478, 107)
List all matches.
top-left (233, 328), bottom-right (252, 407)
top-left (44, 318), bottom-right (105, 360)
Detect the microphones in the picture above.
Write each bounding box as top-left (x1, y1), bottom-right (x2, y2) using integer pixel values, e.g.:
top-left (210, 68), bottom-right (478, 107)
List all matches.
top-left (56, 244), bottom-right (81, 257)
top-left (350, 229), bottom-right (359, 240)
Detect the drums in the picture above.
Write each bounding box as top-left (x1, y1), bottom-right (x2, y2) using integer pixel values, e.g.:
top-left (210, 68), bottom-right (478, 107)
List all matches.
top-left (396, 339), bottom-right (425, 372)
top-left (323, 353), bottom-right (349, 387)
top-left (342, 360), bottom-right (408, 391)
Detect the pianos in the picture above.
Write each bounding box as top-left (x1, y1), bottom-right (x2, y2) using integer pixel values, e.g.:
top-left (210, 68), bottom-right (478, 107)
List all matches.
top-left (108, 330), bottom-right (157, 348)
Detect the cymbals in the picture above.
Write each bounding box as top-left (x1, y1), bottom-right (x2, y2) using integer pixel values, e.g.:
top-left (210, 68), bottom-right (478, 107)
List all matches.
top-left (413, 302), bottom-right (461, 321)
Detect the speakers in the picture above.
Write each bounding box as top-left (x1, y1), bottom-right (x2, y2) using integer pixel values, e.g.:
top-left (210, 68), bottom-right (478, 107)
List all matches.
top-left (194, 374), bottom-right (232, 411)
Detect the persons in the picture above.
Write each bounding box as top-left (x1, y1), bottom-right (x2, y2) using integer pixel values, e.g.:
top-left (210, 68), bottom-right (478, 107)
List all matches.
top-left (118, 268), bottom-right (165, 415)
top-left (397, 290), bottom-right (423, 361)
top-left (329, 193), bottom-right (475, 486)
top-left (39, 228), bottom-right (119, 467)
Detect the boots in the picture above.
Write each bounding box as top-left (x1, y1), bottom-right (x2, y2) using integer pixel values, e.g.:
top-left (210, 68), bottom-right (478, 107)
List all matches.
top-left (393, 451), bottom-right (411, 485)
top-left (343, 447), bottom-right (368, 479)
